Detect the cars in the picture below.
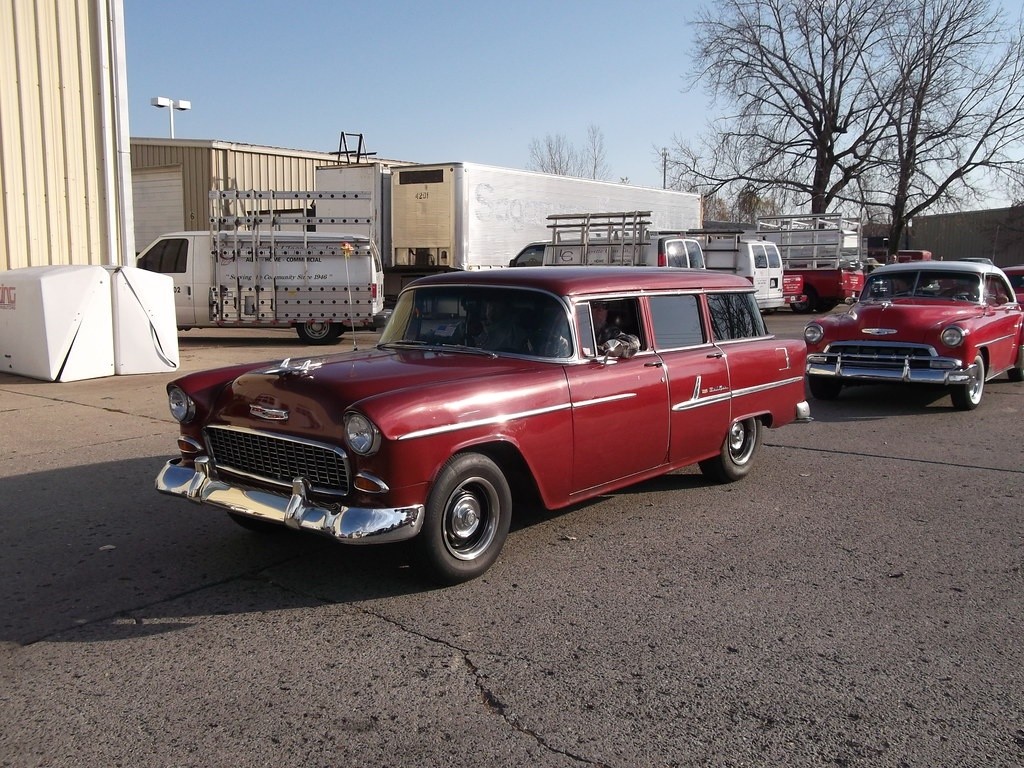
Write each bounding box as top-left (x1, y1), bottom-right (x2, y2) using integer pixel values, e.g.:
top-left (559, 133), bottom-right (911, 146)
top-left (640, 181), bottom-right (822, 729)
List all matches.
top-left (152, 267), bottom-right (815, 589)
top-left (780, 242), bottom-right (1024, 317)
top-left (801, 259), bottom-right (1024, 412)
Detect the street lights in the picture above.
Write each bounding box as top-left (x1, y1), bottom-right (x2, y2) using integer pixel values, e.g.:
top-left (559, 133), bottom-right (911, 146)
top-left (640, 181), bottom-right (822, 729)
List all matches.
top-left (152, 96), bottom-right (192, 138)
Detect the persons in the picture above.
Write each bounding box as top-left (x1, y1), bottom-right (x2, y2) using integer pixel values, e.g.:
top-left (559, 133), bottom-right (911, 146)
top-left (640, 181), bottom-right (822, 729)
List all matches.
top-left (894, 277), bottom-right (913, 290)
top-left (886, 254), bottom-right (898, 295)
top-left (590, 301), bottom-right (641, 358)
top-left (938, 256), bottom-right (943, 261)
top-left (473, 301), bottom-right (527, 350)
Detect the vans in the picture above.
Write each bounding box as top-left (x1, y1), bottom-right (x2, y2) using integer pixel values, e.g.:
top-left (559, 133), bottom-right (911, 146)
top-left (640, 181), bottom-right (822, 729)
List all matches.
top-left (135, 188), bottom-right (393, 346)
top-left (508, 211), bottom-right (707, 269)
top-left (650, 233), bottom-right (787, 315)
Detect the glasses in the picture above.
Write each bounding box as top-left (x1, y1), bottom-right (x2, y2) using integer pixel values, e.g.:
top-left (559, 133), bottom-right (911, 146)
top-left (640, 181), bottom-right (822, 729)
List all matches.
top-left (591, 301), bottom-right (609, 310)
